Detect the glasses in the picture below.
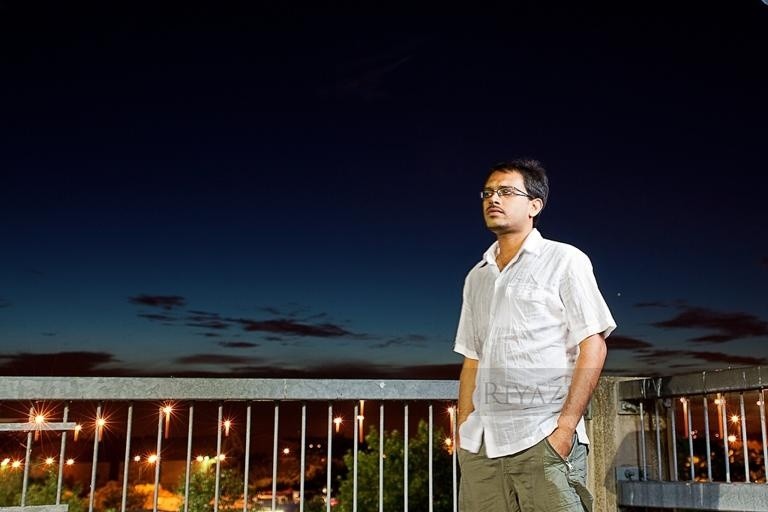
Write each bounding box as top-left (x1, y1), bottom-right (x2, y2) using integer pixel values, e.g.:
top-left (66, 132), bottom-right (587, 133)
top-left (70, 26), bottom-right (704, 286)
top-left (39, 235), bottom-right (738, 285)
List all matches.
top-left (480, 185), bottom-right (533, 198)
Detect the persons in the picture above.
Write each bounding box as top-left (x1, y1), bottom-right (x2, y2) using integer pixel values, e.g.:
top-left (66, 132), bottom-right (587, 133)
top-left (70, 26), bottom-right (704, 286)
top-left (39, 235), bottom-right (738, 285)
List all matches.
top-left (454, 160), bottom-right (617, 511)
